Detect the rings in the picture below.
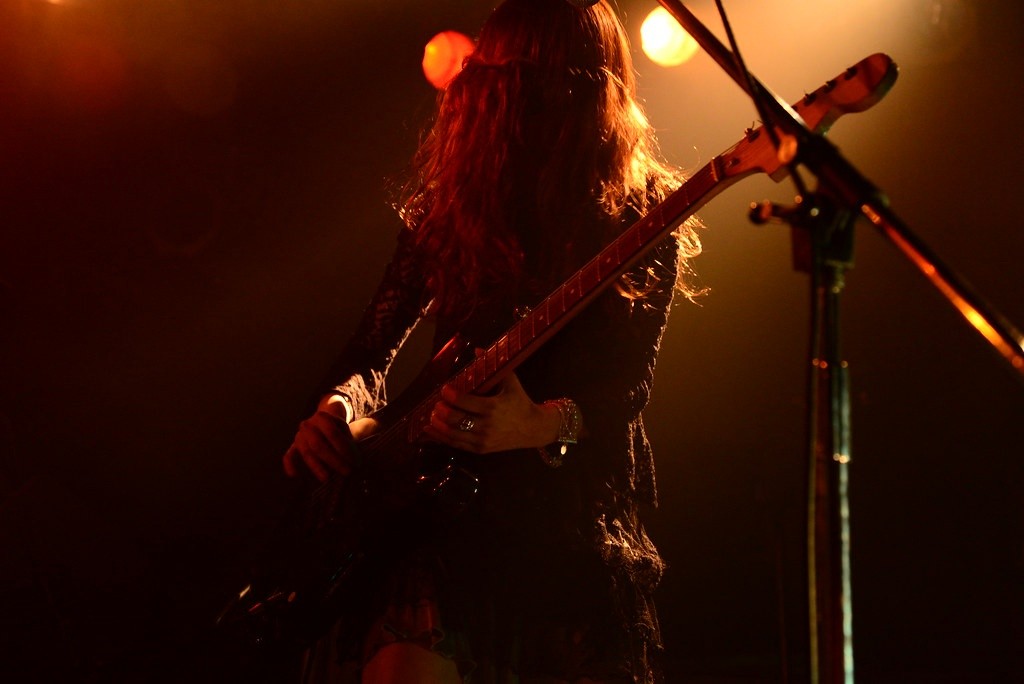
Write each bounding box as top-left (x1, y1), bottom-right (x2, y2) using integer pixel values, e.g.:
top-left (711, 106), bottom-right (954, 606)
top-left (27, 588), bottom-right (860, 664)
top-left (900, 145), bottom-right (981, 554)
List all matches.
top-left (456, 413), bottom-right (476, 433)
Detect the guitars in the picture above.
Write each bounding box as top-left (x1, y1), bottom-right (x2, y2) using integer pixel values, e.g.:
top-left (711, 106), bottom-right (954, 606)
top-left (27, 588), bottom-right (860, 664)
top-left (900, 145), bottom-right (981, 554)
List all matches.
top-left (221, 52), bottom-right (899, 649)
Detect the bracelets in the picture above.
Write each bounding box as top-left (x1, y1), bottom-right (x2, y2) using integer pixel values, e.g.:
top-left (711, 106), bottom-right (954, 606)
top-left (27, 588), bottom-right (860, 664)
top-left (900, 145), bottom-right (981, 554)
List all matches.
top-left (536, 396), bottom-right (579, 469)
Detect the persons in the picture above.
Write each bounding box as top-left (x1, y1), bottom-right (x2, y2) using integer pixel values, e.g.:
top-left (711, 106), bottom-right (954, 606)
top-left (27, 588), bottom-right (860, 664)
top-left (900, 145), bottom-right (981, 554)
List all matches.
top-left (281, 0), bottom-right (706, 684)
